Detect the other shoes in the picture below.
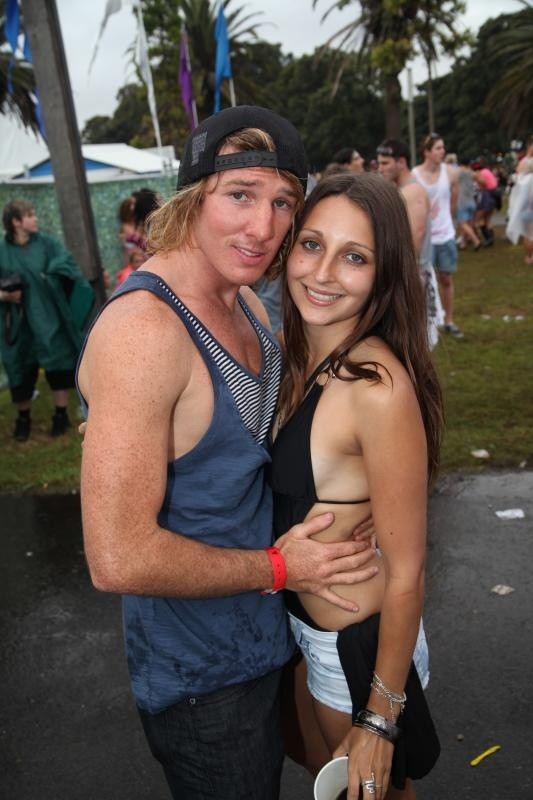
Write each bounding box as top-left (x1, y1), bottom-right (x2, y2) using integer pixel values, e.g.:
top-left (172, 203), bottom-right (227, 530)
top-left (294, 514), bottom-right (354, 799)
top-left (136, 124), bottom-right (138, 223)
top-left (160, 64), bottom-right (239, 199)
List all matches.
top-left (52, 416), bottom-right (68, 434)
top-left (15, 417), bottom-right (30, 440)
top-left (446, 324), bottom-right (463, 337)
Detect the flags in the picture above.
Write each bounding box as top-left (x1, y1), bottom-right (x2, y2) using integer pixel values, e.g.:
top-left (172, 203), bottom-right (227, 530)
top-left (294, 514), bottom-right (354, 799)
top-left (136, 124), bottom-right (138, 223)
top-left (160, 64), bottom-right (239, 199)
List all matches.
top-left (134, 1), bottom-right (233, 131)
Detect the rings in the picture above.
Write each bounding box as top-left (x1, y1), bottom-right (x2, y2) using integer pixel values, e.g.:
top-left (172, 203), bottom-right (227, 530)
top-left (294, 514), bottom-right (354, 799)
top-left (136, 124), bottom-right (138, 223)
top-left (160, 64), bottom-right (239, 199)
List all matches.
top-left (361, 771), bottom-right (376, 793)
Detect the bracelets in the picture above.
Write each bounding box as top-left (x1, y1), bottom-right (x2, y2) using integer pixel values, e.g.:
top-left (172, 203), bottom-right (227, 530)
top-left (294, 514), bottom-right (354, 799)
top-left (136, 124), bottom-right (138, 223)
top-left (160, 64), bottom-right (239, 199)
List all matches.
top-left (369, 671), bottom-right (407, 722)
top-left (353, 707), bottom-right (400, 745)
top-left (262, 545), bottom-right (287, 595)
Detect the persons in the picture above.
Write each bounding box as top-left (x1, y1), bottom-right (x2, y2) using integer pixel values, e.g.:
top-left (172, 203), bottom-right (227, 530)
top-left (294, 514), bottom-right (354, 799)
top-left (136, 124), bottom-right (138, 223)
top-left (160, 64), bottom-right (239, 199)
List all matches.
top-left (76, 106), bottom-right (377, 800)
top-left (0, 199), bottom-right (92, 443)
top-left (101, 131), bottom-right (533, 348)
top-left (79, 173), bottom-right (446, 800)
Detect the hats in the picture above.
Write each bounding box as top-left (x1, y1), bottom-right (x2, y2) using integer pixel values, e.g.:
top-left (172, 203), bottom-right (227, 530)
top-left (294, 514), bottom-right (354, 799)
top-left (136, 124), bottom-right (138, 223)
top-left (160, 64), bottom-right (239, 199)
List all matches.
top-left (177, 105), bottom-right (308, 195)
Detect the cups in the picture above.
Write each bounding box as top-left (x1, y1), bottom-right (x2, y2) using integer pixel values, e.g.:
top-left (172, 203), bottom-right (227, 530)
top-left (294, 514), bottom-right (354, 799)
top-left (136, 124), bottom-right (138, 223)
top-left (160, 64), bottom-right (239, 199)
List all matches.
top-left (313, 756), bottom-right (364, 800)
